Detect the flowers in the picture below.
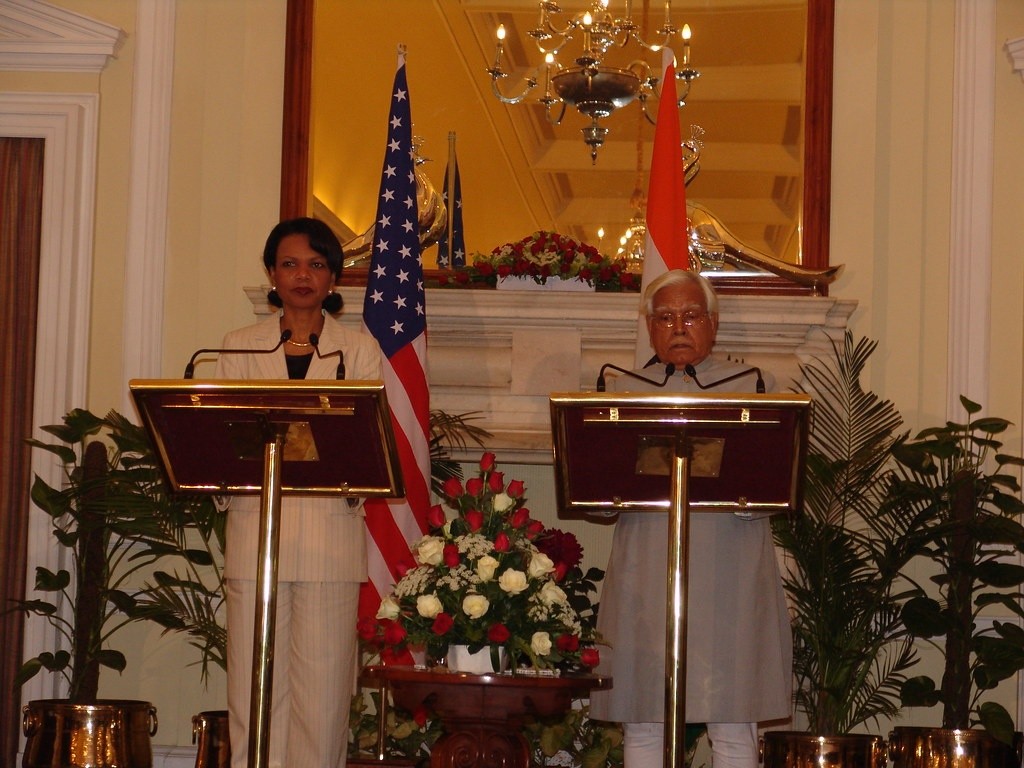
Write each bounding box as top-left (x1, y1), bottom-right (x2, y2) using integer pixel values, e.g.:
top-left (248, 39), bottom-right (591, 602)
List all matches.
top-left (439, 229), bottom-right (642, 293)
top-left (347, 692), bottom-right (442, 768)
top-left (375, 451), bottom-right (614, 677)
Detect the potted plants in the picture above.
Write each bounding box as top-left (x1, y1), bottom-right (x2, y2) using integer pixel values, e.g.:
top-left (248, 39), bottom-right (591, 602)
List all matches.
top-left (0, 408), bottom-right (232, 768)
top-left (757, 329), bottom-right (1024, 768)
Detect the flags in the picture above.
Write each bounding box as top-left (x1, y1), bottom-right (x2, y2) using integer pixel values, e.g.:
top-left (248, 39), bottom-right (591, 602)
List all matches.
top-left (355, 62), bottom-right (447, 728)
top-left (634, 44), bottom-right (690, 371)
top-left (436, 156), bottom-right (466, 270)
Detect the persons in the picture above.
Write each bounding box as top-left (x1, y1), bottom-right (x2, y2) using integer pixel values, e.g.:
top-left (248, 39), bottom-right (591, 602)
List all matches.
top-left (588, 267), bottom-right (793, 768)
top-left (211, 215), bottom-right (383, 768)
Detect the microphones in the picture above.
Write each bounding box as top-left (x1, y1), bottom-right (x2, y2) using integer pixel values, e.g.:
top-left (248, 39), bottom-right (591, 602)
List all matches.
top-left (309, 333), bottom-right (346, 380)
top-left (185, 329), bottom-right (291, 378)
top-left (684, 363), bottom-right (765, 393)
top-left (597, 361), bottom-right (674, 393)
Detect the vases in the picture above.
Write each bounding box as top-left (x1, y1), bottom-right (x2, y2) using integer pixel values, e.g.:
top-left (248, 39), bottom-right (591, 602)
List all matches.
top-left (346, 758), bottom-right (418, 768)
top-left (497, 274), bottom-right (596, 292)
top-left (448, 645), bottom-right (510, 675)
top-left (360, 665), bottom-right (614, 768)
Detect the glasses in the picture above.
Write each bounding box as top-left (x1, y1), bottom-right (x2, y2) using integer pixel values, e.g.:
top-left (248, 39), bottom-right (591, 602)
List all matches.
top-left (653, 311), bottom-right (708, 328)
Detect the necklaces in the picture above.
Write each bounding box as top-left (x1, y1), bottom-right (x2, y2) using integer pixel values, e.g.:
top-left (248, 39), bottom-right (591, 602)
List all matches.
top-left (287, 329), bottom-right (312, 347)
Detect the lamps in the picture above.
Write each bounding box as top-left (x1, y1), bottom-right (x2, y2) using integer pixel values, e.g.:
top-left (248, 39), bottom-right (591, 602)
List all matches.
top-left (486, 0), bottom-right (704, 166)
top-left (608, 0), bottom-right (647, 275)
